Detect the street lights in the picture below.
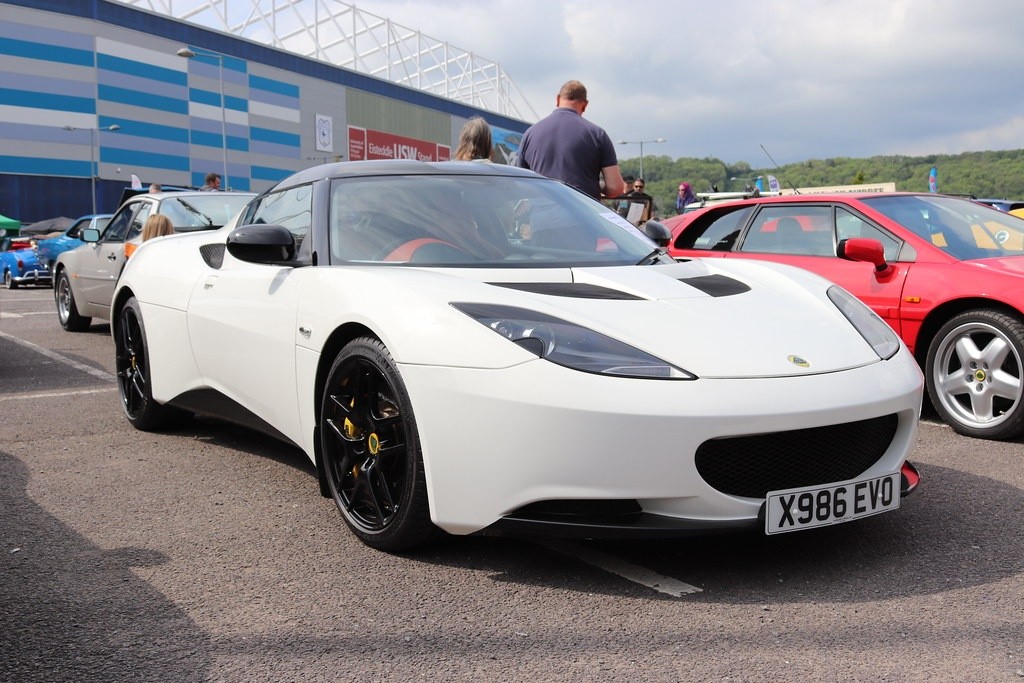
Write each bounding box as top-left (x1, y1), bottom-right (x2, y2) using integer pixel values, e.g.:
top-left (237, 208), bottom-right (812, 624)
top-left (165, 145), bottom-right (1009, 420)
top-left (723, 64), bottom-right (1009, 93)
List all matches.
top-left (617, 138), bottom-right (667, 180)
top-left (62, 124), bottom-right (120, 229)
top-left (178, 48), bottom-right (228, 192)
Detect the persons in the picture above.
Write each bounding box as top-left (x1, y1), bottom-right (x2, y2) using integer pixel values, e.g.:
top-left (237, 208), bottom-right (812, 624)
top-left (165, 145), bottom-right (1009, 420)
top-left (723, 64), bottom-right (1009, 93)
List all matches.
top-left (676, 181), bottom-right (698, 215)
top-left (149, 183), bottom-right (162, 193)
top-left (450, 116), bottom-right (493, 162)
top-left (516, 80), bottom-right (624, 253)
top-left (612, 176), bottom-right (658, 227)
top-left (198, 174), bottom-right (222, 193)
top-left (142, 214), bottom-right (174, 241)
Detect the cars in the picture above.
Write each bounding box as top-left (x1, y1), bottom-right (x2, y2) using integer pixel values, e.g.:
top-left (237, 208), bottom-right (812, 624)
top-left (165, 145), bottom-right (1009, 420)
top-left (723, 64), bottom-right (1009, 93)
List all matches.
top-left (0, 187), bottom-right (198, 289)
top-left (52, 192), bottom-right (258, 333)
top-left (643, 193), bottom-right (1024, 442)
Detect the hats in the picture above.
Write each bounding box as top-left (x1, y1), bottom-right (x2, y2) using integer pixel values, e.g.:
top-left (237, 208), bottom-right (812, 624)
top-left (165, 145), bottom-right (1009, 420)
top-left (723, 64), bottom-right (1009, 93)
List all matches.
top-left (623, 176), bottom-right (634, 183)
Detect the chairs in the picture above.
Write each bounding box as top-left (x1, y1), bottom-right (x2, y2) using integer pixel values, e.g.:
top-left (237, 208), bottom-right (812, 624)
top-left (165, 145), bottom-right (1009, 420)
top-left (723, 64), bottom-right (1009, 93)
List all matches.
top-left (885, 202), bottom-right (933, 243)
top-left (774, 217), bottom-right (804, 243)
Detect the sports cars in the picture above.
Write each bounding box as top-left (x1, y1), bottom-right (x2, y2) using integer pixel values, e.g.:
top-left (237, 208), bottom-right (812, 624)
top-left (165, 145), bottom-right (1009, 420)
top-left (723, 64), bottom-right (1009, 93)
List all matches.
top-left (110, 160), bottom-right (925, 551)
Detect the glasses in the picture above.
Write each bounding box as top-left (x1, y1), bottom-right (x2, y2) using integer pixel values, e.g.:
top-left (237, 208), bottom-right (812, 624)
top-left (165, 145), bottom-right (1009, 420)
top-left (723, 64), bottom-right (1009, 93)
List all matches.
top-left (678, 189), bottom-right (684, 191)
top-left (635, 185), bottom-right (643, 188)
